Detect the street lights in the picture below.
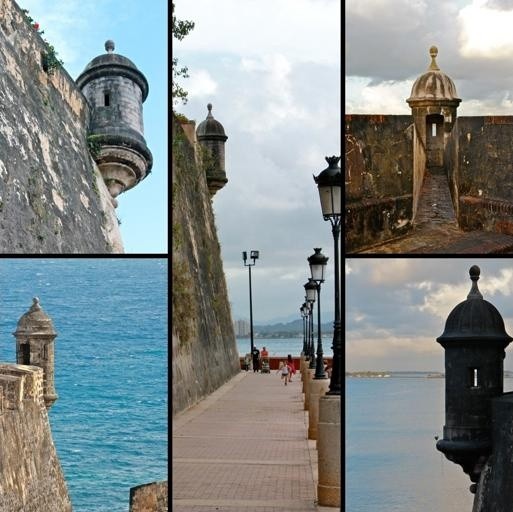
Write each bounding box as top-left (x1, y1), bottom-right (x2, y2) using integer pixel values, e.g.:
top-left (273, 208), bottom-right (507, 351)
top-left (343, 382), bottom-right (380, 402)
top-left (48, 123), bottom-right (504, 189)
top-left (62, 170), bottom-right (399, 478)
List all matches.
top-left (299, 248), bottom-right (329, 380)
top-left (243, 249), bottom-right (259, 351)
top-left (314, 156), bottom-right (341, 395)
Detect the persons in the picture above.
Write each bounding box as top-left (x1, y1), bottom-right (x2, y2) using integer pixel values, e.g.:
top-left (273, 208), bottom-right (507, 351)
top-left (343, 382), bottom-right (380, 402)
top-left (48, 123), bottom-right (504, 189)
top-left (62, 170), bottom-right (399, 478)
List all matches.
top-left (244, 353), bottom-right (251, 371)
top-left (276, 360), bottom-right (291, 386)
top-left (251, 347), bottom-right (259, 373)
top-left (261, 347), bottom-right (268, 369)
top-left (287, 354), bottom-right (296, 378)
